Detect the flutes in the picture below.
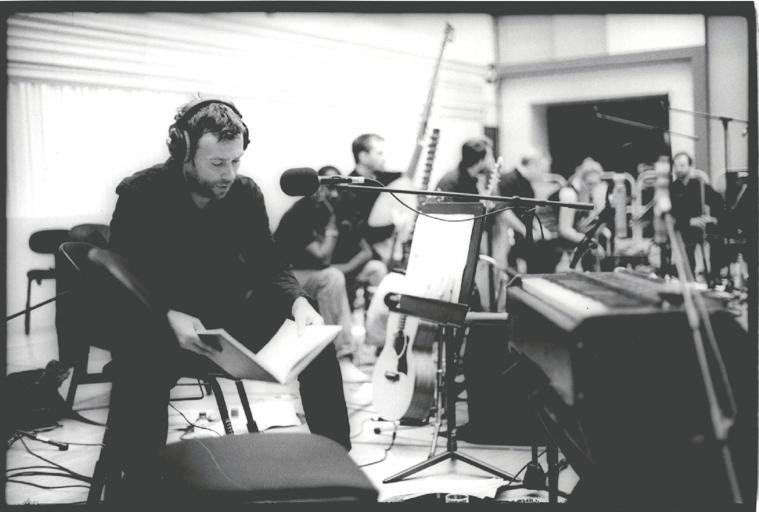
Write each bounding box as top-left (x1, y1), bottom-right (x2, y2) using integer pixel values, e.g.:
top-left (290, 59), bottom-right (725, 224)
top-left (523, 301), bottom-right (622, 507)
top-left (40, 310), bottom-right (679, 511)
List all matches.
top-left (484, 155), bottom-right (503, 195)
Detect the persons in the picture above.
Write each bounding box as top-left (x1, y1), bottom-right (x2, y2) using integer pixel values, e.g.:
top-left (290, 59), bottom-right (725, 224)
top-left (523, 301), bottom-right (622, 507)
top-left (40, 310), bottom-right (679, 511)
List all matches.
top-left (270, 133), bottom-right (419, 360)
top-left (430, 136), bottom-right (727, 314)
top-left (104, 96), bottom-right (351, 499)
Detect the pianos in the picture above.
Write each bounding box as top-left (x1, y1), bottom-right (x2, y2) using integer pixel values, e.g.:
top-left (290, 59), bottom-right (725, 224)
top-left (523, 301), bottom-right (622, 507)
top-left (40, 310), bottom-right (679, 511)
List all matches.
top-left (501, 266), bottom-right (749, 509)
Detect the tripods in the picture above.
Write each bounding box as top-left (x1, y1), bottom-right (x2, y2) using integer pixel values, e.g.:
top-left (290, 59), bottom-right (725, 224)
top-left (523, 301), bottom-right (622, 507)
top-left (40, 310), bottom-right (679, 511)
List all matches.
top-left (492, 422), bottom-right (570, 500)
top-left (383, 326), bottom-right (522, 482)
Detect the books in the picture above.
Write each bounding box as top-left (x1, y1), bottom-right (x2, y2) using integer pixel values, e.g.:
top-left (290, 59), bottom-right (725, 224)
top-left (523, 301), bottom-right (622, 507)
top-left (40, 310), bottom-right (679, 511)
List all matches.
top-left (196, 318), bottom-right (344, 385)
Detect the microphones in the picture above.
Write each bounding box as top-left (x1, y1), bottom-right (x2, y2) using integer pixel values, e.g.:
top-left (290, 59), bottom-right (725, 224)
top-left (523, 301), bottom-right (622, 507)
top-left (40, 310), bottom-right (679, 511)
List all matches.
top-left (593, 104), bottom-right (601, 119)
top-left (659, 97), bottom-right (667, 114)
top-left (280, 167), bottom-right (371, 196)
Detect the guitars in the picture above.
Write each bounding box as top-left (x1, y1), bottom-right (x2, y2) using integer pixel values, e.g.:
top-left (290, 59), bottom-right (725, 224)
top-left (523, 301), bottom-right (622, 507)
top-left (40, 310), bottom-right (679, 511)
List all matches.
top-left (372, 191), bottom-right (456, 425)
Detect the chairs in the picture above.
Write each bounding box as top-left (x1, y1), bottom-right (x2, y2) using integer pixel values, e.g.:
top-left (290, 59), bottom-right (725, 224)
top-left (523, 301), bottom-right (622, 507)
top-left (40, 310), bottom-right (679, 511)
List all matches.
top-left (23, 222), bottom-right (378, 512)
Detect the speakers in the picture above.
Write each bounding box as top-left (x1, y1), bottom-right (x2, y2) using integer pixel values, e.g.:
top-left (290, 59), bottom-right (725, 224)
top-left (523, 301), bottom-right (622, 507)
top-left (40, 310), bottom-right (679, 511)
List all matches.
top-left (104, 432), bottom-right (380, 511)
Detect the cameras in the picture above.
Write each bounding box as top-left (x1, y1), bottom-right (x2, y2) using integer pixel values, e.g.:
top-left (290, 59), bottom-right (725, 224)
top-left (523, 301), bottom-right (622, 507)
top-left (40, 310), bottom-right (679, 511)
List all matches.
top-left (167, 97), bottom-right (250, 164)
